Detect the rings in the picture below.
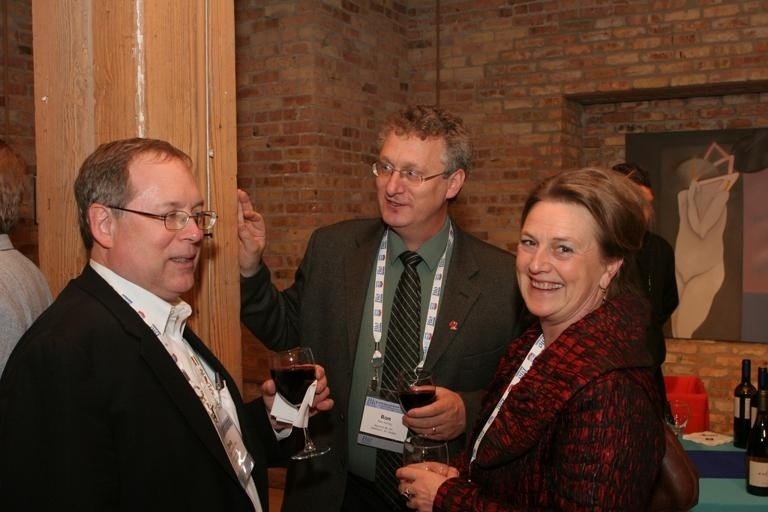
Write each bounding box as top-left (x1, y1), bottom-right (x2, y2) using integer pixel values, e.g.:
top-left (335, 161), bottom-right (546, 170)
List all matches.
top-left (433, 426), bottom-right (437, 434)
top-left (400, 489), bottom-right (412, 503)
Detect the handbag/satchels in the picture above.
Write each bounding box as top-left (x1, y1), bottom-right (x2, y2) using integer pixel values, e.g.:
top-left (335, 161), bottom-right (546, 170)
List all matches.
top-left (653, 422), bottom-right (699, 512)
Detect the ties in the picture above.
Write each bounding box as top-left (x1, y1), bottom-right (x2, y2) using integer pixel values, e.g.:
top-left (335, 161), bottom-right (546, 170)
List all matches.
top-left (377, 251), bottom-right (422, 504)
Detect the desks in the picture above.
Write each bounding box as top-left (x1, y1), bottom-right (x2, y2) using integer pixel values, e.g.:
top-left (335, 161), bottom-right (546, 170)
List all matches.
top-left (679, 435), bottom-right (768, 511)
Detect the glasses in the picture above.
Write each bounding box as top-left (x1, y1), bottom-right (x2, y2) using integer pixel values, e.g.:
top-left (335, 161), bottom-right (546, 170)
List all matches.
top-left (373, 162), bottom-right (452, 185)
top-left (107, 205), bottom-right (218, 231)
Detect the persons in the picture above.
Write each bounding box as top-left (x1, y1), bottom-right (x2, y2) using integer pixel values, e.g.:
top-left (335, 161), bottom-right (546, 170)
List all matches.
top-left (613, 164), bottom-right (679, 423)
top-left (395, 164), bottom-right (665, 510)
top-left (235, 104), bottom-right (539, 512)
top-left (0, 222), bottom-right (53, 382)
top-left (0, 138), bottom-right (335, 512)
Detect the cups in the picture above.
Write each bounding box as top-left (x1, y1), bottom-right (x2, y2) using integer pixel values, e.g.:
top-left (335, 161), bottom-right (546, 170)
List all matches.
top-left (403, 435), bottom-right (450, 480)
top-left (397, 368), bottom-right (436, 417)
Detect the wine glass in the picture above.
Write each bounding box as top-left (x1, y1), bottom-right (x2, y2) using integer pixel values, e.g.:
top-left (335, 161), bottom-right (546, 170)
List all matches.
top-left (266, 344), bottom-right (332, 461)
top-left (664, 401), bottom-right (689, 449)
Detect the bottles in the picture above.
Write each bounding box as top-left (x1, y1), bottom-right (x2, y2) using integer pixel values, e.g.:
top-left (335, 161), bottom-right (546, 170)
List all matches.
top-left (745, 388), bottom-right (768, 498)
top-left (732, 358), bottom-right (768, 451)
